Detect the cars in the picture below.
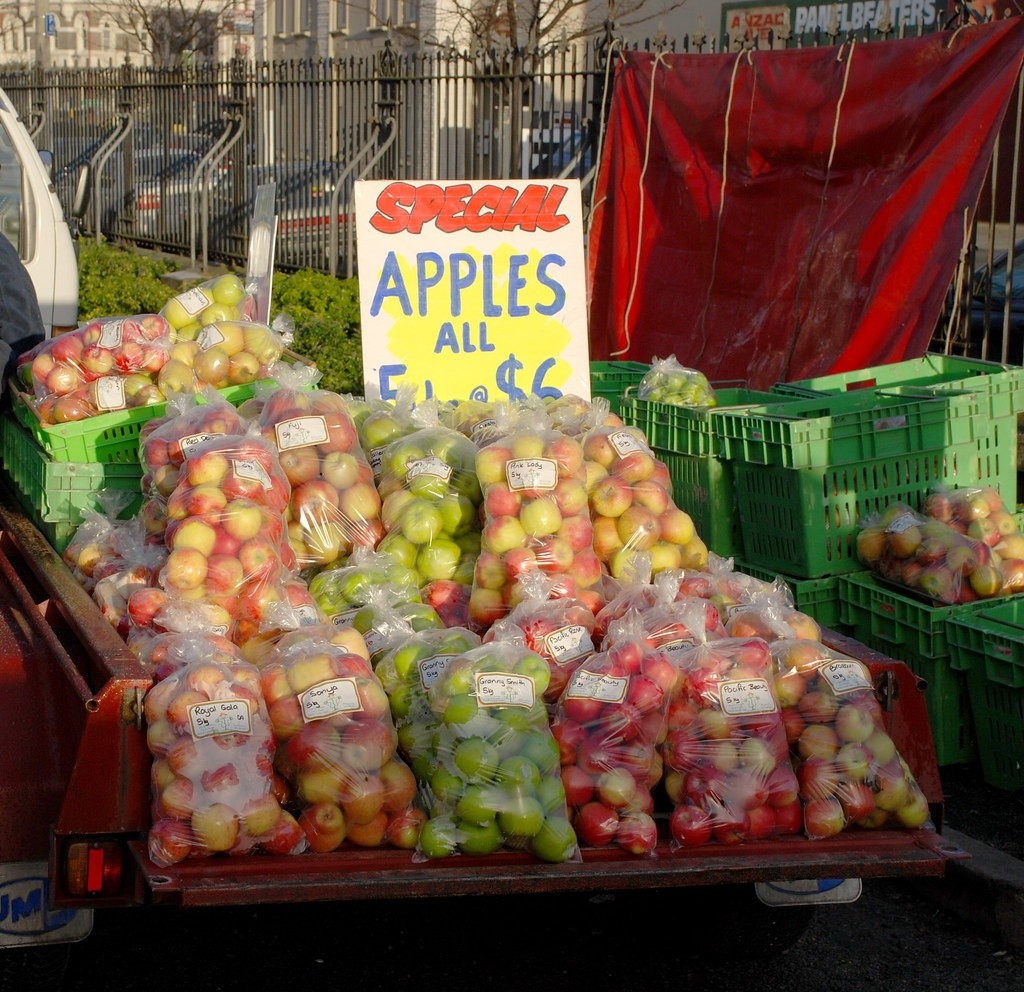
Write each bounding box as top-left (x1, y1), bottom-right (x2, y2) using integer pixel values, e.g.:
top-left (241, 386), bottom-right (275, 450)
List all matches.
top-left (939, 236), bottom-right (1024, 365)
top-left (40, 135), bottom-right (365, 268)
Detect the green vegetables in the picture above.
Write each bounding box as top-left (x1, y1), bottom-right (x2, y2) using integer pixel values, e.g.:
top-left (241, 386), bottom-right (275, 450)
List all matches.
top-left (75, 237), bottom-right (367, 392)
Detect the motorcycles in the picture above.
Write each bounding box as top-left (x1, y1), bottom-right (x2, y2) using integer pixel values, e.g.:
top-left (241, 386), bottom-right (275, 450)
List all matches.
top-left (0, 491), bottom-right (974, 964)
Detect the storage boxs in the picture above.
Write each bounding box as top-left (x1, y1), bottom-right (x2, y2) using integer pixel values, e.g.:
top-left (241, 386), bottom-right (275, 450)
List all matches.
top-left (0, 370), bottom-right (314, 554)
top-left (592, 351), bottom-right (1024, 794)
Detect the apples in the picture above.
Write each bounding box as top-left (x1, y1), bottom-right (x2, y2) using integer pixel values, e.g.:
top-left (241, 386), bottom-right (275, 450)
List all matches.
top-left (18, 274), bottom-right (1024, 860)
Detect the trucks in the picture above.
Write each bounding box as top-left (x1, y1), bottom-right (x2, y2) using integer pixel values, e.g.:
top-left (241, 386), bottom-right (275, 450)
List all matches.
top-left (0, 89), bottom-right (94, 343)
